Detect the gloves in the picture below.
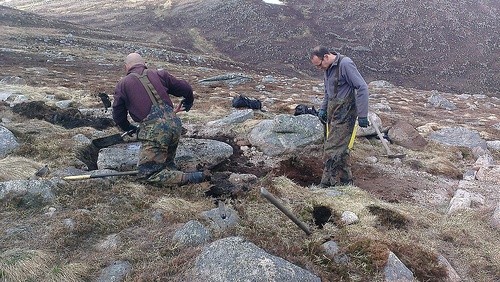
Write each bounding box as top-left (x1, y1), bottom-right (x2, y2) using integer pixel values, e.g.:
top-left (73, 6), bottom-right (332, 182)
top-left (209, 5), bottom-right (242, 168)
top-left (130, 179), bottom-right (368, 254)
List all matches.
top-left (358, 117), bottom-right (370, 129)
top-left (127, 124), bottom-right (138, 138)
top-left (318, 110), bottom-right (327, 124)
top-left (181, 99), bottom-right (193, 112)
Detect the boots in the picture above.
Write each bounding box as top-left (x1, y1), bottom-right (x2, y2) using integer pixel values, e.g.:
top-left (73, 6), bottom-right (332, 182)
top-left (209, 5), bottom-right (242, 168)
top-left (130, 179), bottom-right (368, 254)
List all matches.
top-left (339, 176), bottom-right (353, 186)
top-left (318, 161), bottom-right (337, 189)
top-left (186, 172), bottom-right (214, 184)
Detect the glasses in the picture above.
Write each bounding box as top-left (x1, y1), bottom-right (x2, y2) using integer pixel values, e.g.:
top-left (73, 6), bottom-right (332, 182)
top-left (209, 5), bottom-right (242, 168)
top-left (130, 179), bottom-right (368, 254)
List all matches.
top-left (314, 60), bottom-right (323, 68)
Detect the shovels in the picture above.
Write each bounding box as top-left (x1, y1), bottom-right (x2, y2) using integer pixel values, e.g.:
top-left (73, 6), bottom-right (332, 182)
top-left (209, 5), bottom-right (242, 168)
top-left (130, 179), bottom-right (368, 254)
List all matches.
top-left (368, 114), bottom-right (408, 159)
top-left (91, 99), bottom-right (191, 149)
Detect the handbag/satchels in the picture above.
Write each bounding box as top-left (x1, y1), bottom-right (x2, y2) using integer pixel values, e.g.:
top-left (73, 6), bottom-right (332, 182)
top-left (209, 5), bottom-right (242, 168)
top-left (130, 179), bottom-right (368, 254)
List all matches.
top-left (232, 94), bottom-right (262, 110)
top-left (294, 104), bottom-right (319, 116)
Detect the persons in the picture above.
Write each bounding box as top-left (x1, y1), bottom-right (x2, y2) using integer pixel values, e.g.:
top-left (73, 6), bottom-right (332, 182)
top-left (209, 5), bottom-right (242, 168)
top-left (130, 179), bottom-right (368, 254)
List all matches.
top-left (309, 46), bottom-right (370, 188)
top-left (111, 53), bottom-right (213, 187)
